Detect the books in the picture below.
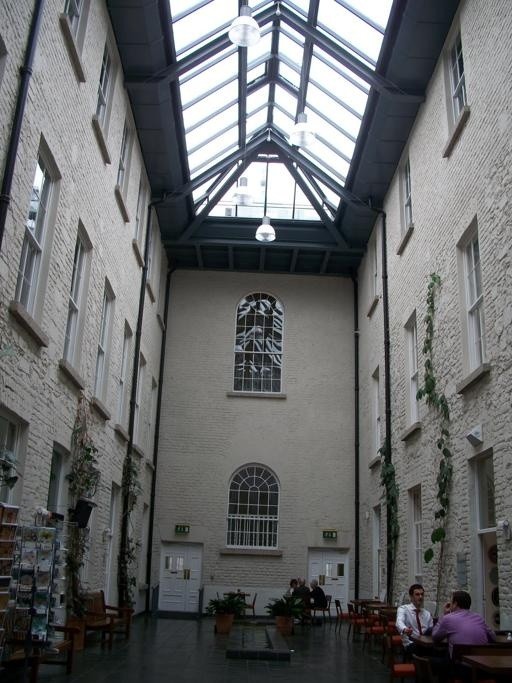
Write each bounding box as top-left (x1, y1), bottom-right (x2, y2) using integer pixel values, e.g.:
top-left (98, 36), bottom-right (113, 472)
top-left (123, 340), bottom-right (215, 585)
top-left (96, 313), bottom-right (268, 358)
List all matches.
top-left (0, 500), bottom-right (21, 635)
top-left (13, 526), bottom-right (58, 636)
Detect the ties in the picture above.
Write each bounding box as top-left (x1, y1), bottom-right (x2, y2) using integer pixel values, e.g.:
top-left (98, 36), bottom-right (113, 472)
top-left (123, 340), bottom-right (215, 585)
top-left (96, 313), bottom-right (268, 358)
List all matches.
top-left (414, 609), bottom-right (422, 634)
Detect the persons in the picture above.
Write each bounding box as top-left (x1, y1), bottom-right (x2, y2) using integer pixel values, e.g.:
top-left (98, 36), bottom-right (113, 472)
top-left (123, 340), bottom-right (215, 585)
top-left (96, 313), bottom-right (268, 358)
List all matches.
top-left (309, 579), bottom-right (327, 608)
top-left (292, 575), bottom-right (311, 626)
top-left (395, 584), bottom-right (434, 663)
top-left (286, 578), bottom-right (298, 594)
top-left (431, 590), bottom-right (496, 663)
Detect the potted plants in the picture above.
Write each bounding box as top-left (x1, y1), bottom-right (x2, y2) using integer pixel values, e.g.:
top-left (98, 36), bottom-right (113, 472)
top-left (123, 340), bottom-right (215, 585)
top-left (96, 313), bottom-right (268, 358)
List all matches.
top-left (264, 598), bottom-right (304, 633)
top-left (205, 598), bottom-right (247, 634)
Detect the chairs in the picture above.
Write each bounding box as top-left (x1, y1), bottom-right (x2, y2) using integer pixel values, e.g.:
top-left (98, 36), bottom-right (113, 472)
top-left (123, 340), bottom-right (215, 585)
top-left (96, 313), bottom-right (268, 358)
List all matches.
top-left (334, 597), bottom-right (512, 681)
top-left (0, 607), bottom-right (83, 682)
top-left (311, 595), bottom-right (334, 624)
top-left (72, 589), bottom-right (135, 654)
top-left (216, 590), bottom-right (258, 616)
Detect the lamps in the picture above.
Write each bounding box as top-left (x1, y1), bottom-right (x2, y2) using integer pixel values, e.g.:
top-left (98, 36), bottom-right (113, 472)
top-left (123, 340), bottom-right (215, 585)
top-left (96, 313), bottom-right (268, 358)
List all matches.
top-left (252, 144), bottom-right (278, 244)
top-left (288, 43), bottom-right (318, 148)
top-left (224, 1), bottom-right (262, 49)
